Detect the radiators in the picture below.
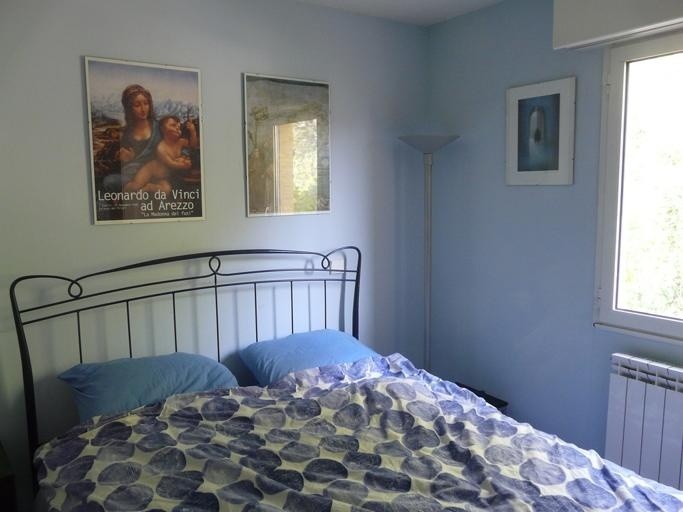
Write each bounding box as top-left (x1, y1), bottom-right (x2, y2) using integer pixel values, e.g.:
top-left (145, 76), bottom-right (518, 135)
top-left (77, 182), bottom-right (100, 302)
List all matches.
top-left (603, 349), bottom-right (682, 491)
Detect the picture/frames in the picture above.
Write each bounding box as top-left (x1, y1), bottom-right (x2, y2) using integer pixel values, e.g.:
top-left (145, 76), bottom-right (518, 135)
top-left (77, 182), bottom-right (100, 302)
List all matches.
top-left (84, 54), bottom-right (206, 223)
top-left (503, 75), bottom-right (577, 188)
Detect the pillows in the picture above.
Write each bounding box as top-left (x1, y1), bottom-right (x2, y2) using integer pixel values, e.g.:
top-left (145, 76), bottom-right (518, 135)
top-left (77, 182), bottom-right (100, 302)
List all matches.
top-left (240, 328), bottom-right (380, 388)
top-left (58, 351), bottom-right (240, 428)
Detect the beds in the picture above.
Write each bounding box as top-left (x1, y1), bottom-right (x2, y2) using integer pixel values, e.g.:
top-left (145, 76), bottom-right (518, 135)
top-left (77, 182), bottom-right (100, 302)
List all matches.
top-left (6, 245), bottom-right (682, 511)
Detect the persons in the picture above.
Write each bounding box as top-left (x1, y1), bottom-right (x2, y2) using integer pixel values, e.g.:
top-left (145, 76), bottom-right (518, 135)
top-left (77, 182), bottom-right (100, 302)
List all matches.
top-left (103, 84), bottom-right (190, 200)
top-left (112, 115), bottom-right (196, 206)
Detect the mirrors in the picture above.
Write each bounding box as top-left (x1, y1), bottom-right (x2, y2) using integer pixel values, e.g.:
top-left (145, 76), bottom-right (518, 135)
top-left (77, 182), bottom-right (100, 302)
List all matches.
top-left (241, 72), bottom-right (333, 218)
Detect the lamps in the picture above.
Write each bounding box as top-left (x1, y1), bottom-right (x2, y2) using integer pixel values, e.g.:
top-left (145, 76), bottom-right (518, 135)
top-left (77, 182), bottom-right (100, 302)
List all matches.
top-left (395, 133), bottom-right (461, 372)
